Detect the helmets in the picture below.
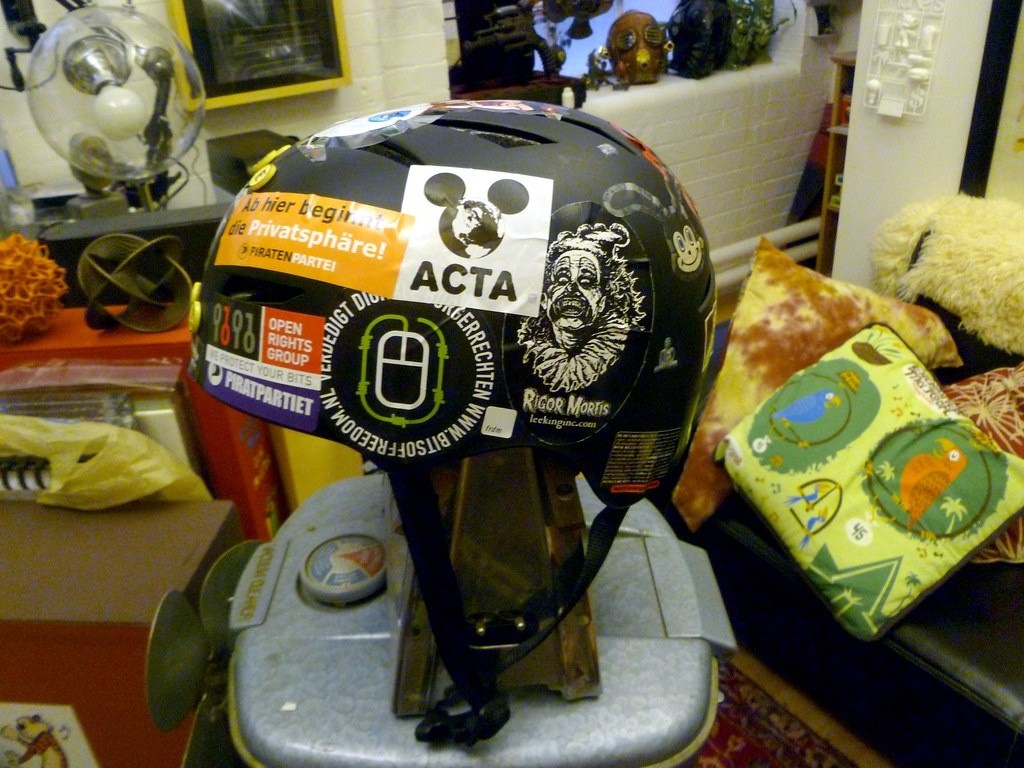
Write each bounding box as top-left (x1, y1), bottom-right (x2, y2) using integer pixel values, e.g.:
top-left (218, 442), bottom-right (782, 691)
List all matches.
top-left (188, 98), bottom-right (718, 508)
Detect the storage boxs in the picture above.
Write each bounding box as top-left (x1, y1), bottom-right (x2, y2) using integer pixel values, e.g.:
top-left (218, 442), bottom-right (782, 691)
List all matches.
top-left (224, 475), bottom-right (738, 768)
top-left (0, 305), bottom-right (282, 541)
top-left (268, 422), bottom-right (364, 513)
top-left (0, 499), bottom-right (245, 768)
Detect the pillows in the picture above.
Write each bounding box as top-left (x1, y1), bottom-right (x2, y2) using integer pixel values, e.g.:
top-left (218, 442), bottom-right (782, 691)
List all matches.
top-left (672, 236), bottom-right (963, 534)
top-left (940, 360), bottom-right (1024, 563)
top-left (711, 321), bottom-right (1024, 642)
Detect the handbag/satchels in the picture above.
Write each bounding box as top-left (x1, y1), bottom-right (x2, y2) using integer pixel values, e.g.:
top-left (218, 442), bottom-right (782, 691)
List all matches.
top-left (0, 411), bottom-right (218, 513)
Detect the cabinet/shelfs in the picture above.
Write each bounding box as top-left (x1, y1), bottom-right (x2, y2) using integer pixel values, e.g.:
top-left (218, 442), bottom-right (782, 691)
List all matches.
top-left (815, 50), bottom-right (856, 276)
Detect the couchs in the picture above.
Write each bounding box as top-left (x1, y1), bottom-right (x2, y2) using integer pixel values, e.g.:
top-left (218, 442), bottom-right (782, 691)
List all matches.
top-left (660, 190), bottom-right (1024, 768)
